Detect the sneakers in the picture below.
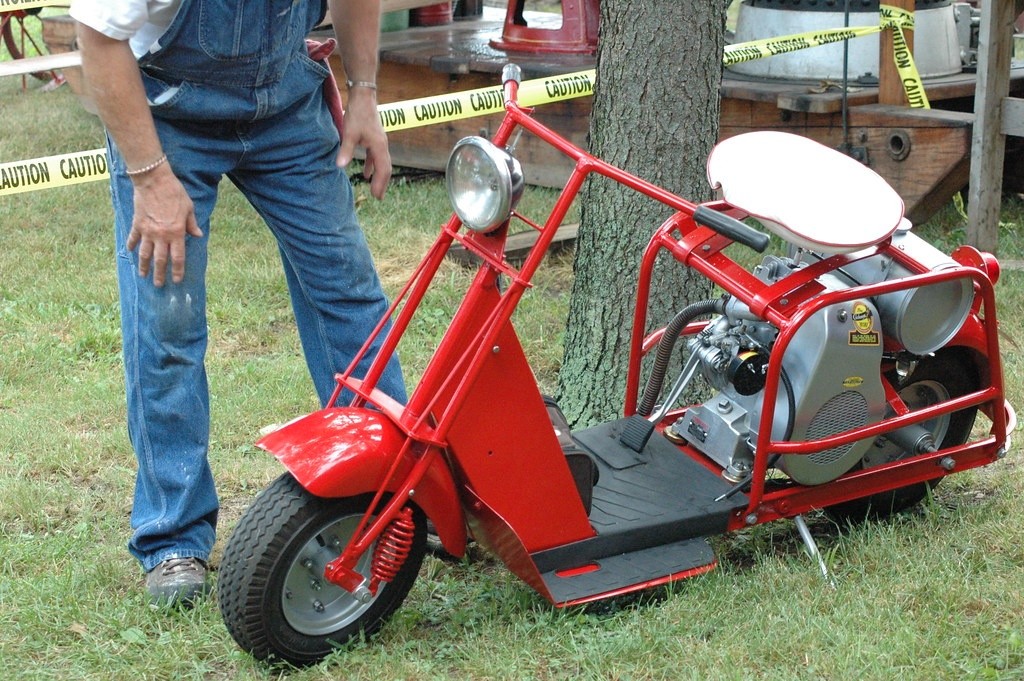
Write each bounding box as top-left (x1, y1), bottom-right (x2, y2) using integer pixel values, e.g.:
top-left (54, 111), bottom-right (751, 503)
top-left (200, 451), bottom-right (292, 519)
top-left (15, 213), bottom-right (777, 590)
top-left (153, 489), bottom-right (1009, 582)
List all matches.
top-left (147, 558), bottom-right (208, 607)
top-left (426, 521), bottom-right (479, 554)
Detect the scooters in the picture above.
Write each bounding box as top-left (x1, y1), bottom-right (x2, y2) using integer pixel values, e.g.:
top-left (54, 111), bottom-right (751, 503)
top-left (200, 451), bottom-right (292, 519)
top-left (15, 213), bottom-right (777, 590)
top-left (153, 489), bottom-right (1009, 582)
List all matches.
top-left (213, 60), bottom-right (1017, 668)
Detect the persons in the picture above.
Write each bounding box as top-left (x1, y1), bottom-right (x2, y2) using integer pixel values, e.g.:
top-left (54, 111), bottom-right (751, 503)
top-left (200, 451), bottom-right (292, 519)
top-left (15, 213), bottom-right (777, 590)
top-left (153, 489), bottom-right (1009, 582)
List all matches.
top-left (63, 1), bottom-right (486, 615)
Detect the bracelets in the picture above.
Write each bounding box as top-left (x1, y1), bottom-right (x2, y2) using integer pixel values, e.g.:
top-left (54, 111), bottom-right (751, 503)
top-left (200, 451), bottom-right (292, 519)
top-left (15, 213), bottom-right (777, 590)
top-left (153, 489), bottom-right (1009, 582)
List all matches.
top-left (346, 78), bottom-right (381, 91)
top-left (124, 152), bottom-right (169, 176)
